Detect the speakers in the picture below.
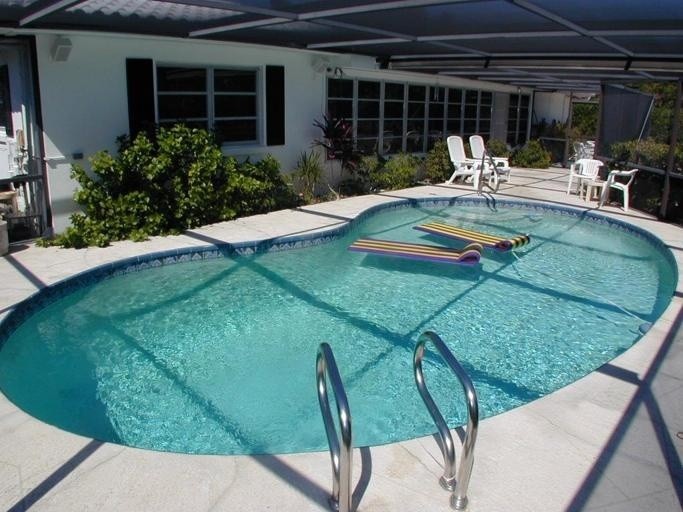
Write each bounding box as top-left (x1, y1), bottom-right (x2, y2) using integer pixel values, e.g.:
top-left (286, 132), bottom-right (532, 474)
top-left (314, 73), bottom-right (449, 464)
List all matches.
top-left (50, 38), bottom-right (71, 62)
top-left (312, 56), bottom-right (329, 74)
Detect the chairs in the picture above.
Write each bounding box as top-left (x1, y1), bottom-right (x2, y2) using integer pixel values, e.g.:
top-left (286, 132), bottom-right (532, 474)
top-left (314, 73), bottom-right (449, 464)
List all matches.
top-left (596, 169), bottom-right (638, 212)
top-left (566, 159), bottom-right (604, 200)
top-left (445, 136), bottom-right (492, 190)
top-left (465, 135), bottom-right (510, 184)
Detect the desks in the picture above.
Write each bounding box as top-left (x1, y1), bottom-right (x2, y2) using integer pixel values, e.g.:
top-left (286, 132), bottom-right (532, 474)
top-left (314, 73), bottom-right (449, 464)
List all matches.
top-left (579, 179), bottom-right (611, 205)
